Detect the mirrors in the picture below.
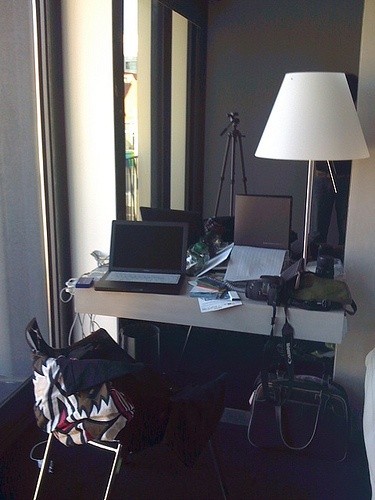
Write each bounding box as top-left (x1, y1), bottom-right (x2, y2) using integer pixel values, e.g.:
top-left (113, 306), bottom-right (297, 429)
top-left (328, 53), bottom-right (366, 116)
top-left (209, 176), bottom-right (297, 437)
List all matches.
top-left (111, 0), bottom-right (366, 280)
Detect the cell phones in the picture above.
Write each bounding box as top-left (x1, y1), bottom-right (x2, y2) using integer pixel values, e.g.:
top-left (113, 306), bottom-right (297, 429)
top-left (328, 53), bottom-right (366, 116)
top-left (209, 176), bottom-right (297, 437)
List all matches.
top-left (76, 278), bottom-right (93, 287)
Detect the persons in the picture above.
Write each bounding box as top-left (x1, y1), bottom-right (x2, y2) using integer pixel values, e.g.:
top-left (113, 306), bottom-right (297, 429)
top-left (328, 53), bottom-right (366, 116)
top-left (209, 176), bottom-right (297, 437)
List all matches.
top-left (311, 73), bottom-right (358, 251)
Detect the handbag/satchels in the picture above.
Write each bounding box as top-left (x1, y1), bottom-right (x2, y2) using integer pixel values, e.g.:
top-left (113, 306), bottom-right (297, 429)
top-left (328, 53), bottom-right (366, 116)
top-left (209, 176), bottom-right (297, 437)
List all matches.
top-left (280, 271), bottom-right (357, 315)
top-left (242, 370), bottom-right (353, 464)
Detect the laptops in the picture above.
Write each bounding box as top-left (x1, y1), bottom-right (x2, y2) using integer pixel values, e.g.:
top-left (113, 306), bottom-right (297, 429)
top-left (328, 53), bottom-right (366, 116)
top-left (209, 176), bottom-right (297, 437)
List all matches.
top-left (94, 219), bottom-right (189, 296)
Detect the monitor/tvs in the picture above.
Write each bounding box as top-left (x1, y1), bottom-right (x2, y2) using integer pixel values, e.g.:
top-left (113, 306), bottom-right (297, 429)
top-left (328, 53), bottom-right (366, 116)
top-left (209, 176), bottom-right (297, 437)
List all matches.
top-left (140, 206), bottom-right (204, 247)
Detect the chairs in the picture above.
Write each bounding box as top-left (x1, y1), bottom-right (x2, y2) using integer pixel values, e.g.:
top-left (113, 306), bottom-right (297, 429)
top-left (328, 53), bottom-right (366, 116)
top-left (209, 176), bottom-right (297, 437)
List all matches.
top-left (25, 318), bottom-right (177, 500)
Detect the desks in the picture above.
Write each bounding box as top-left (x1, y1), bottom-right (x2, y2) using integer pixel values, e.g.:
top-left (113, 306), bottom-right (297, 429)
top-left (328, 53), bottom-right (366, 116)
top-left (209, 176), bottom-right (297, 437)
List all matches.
top-left (73, 277), bottom-right (345, 412)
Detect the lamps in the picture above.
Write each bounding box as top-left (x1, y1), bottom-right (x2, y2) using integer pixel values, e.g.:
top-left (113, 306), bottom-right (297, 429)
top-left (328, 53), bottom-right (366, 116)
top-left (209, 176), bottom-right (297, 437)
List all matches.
top-left (254, 72), bottom-right (370, 272)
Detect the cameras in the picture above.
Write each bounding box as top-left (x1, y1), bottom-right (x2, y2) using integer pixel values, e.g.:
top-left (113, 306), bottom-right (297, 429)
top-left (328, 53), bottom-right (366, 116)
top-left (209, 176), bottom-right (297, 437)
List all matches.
top-left (245, 276), bottom-right (293, 305)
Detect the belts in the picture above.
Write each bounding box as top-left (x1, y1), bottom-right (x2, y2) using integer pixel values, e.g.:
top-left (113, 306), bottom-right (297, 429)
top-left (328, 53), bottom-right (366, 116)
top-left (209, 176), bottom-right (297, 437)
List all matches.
top-left (314, 169), bottom-right (350, 178)
